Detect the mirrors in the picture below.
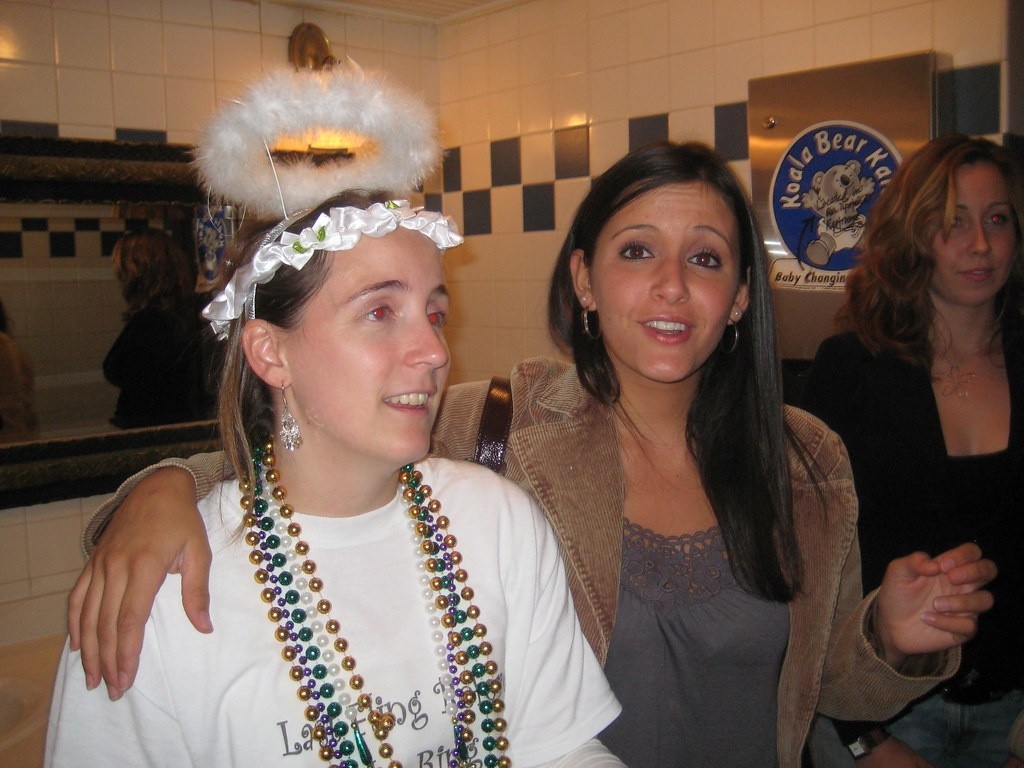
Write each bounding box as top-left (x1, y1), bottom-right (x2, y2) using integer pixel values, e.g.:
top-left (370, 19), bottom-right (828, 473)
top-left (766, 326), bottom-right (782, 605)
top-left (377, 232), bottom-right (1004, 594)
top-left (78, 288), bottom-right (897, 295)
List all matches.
top-left (0, 135), bottom-right (357, 511)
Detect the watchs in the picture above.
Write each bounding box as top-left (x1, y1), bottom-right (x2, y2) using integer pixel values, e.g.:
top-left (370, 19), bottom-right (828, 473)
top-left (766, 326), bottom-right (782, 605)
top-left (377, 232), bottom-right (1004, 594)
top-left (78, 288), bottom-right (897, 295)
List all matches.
top-left (847, 725), bottom-right (890, 760)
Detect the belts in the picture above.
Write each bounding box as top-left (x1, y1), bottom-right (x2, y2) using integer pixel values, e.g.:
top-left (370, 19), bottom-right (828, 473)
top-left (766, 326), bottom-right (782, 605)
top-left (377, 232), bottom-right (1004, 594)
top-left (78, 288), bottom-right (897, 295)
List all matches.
top-left (942, 670), bottom-right (1015, 704)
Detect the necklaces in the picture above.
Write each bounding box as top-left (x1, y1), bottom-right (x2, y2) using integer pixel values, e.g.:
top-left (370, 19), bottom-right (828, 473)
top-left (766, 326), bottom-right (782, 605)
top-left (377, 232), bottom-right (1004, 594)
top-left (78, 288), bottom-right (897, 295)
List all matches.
top-left (931, 347), bottom-right (997, 398)
top-left (239, 436), bottom-right (513, 768)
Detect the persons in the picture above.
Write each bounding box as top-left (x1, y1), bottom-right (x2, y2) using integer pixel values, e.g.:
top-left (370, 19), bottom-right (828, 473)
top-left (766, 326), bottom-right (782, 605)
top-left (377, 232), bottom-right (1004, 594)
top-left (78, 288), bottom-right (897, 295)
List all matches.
top-left (68, 140), bottom-right (997, 768)
top-left (0, 297), bottom-right (39, 442)
top-left (101, 227), bottom-right (230, 430)
top-left (46, 71), bottom-right (620, 768)
top-left (803, 134), bottom-right (1024, 768)
